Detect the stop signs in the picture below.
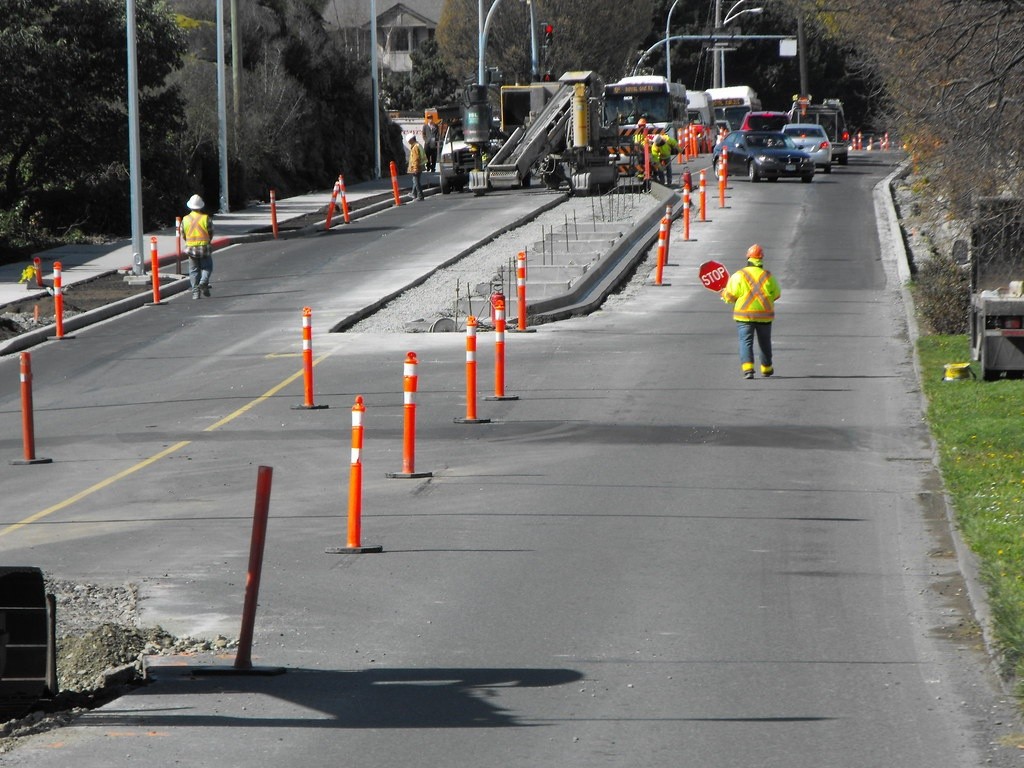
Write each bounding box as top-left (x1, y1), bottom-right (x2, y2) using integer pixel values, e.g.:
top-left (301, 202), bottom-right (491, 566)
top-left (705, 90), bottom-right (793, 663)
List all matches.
top-left (700, 260), bottom-right (731, 292)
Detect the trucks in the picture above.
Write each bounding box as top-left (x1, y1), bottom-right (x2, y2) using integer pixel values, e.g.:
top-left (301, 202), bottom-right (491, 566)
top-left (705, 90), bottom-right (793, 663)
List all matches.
top-left (789, 93), bottom-right (853, 160)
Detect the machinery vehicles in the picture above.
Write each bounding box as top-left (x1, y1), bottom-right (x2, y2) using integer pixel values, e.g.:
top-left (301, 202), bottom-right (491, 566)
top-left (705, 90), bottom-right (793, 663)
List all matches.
top-left (438, 68), bottom-right (624, 198)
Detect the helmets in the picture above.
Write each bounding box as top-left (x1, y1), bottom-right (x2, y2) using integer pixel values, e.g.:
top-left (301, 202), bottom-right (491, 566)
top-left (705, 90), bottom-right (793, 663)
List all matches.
top-left (405, 133), bottom-right (415, 142)
top-left (187, 195), bottom-right (205, 209)
top-left (652, 134), bottom-right (663, 145)
top-left (427, 114), bottom-right (433, 118)
top-left (638, 119), bottom-right (646, 125)
top-left (746, 244), bottom-right (763, 259)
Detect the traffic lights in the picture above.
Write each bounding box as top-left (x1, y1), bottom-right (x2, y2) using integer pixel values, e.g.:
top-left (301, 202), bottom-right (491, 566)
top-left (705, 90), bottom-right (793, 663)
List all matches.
top-left (543, 74), bottom-right (555, 82)
top-left (541, 22), bottom-right (554, 49)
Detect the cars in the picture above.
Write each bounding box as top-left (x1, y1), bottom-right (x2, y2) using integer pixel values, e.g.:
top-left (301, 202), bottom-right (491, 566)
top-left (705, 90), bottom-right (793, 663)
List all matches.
top-left (709, 130), bottom-right (815, 183)
top-left (782, 124), bottom-right (839, 168)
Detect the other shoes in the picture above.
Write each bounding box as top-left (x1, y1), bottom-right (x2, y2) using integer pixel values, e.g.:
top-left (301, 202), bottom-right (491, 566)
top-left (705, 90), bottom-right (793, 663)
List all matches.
top-left (427, 167), bottom-right (430, 171)
top-left (199, 283), bottom-right (210, 296)
top-left (408, 193), bottom-right (415, 198)
top-left (743, 373), bottom-right (754, 379)
top-left (192, 294), bottom-right (200, 300)
top-left (412, 198), bottom-right (420, 201)
top-left (762, 368), bottom-right (774, 377)
top-left (431, 168), bottom-right (435, 172)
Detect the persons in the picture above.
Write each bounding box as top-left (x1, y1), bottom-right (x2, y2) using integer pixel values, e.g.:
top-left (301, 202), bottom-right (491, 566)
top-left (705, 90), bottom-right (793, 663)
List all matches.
top-left (655, 104), bottom-right (665, 114)
top-left (423, 115), bottom-right (439, 172)
top-left (767, 139), bottom-right (775, 147)
top-left (181, 194), bottom-right (215, 301)
top-left (719, 243), bottom-right (780, 379)
top-left (651, 133), bottom-right (685, 186)
top-left (406, 133), bottom-right (424, 201)
top-left (633, 118), bottom-right (652, 178)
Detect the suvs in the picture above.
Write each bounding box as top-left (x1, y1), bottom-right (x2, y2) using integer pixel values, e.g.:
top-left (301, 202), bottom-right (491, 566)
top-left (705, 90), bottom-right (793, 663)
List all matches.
top-left (741, 109), bottom-right (796, 131)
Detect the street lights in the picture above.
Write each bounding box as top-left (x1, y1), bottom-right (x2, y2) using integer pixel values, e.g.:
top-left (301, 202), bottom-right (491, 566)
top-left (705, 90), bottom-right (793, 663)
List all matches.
top-left (723, 0), bottom-right (765, 90)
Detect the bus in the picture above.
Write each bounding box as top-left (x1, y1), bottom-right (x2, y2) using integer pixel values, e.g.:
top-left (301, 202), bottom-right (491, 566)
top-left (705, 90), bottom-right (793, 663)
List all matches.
top-left (705, 87), bottom-right (762, 131)
top-left (603, 76), bottom-right (689, 155)
top-left (686, 90), bottom-right (713, 128)
